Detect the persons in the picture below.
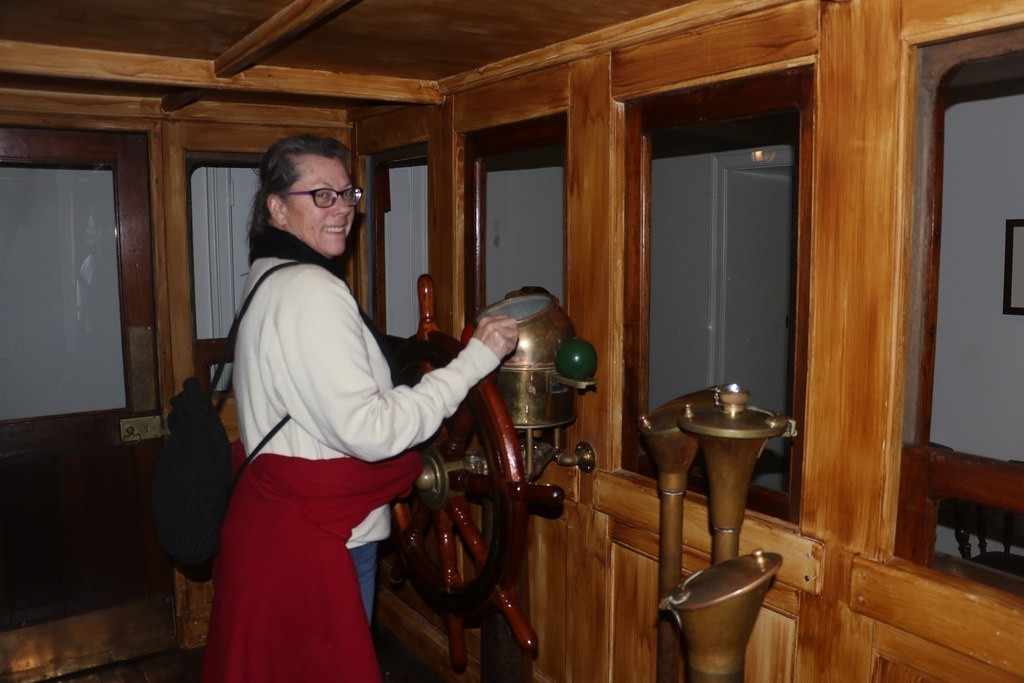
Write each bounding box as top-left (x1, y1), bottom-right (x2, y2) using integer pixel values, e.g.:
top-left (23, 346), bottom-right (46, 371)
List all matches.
top-left (204, 134), bottom-right (519, 683)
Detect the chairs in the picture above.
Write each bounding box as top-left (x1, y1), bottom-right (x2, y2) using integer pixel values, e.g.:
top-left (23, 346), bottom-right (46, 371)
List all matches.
top-left (953, 499), bottom-right (1023, 576)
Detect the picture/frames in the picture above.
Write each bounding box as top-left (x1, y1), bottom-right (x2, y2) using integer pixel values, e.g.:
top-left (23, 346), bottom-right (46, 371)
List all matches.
top-left (1003, 218), bottom-right (1024, 316)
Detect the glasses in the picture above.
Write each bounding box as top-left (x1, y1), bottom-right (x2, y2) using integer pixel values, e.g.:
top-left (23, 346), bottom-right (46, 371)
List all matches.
top-left (279, 188), bottom-right (363, 209)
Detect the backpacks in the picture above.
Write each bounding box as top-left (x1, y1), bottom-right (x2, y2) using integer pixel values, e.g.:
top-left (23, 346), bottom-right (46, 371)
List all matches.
top-left (149, 261), bottom-right (334, 582)
